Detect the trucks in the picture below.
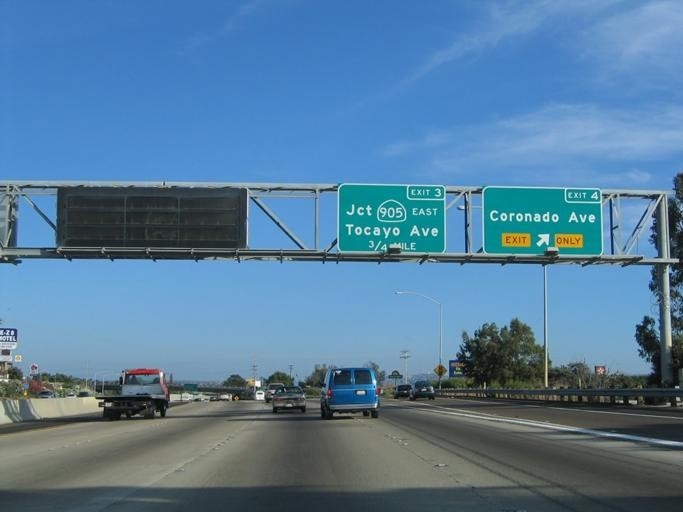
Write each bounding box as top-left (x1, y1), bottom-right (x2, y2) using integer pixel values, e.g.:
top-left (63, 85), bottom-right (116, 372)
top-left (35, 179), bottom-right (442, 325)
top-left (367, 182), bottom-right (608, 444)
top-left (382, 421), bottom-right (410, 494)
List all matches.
top-left (96, 368), bottom-right (170, 419)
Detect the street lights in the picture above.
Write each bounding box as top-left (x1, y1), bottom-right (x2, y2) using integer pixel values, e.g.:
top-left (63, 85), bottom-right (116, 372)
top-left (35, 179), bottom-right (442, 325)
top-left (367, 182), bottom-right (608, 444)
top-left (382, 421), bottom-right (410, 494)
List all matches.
top-left (458, 206), bottom-right (547, 388)
top-left (392, 292), bottom-right (442, 390)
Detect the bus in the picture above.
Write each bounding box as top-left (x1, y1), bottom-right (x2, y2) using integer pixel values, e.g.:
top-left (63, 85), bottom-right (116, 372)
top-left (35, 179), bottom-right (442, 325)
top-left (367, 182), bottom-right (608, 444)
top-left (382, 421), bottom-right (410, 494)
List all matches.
top-left (253, 391), bottom-right (265, 401)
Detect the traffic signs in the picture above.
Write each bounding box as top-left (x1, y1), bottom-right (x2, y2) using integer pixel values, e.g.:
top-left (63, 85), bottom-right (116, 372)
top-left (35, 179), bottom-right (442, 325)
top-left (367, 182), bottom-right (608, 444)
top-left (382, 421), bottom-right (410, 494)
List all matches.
top-left (482, 186), bottom-right (604, 258)
top-left (337, 183), bottom-right (447, 256)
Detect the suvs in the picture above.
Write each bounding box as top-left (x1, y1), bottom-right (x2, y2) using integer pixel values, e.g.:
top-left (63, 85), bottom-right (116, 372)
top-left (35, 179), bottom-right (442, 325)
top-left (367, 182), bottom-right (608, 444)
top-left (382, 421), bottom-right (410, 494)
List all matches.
top-left (265, 383), bottom-right (286, 403)
top-left (320, 367), bottom-right (379, 418)
top-left (393, 380), bottom-right (434, 401)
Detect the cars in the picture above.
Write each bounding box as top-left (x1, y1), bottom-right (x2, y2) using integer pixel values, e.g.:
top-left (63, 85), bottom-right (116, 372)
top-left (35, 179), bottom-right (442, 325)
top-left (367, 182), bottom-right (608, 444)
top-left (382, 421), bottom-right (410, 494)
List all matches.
top-left (66, 391), bottom-right (88, 398)
top-left (39, 391), bottom-right (54, 399)
top-left (210, 392), bottom-right (232, 401)
top-left (272, 386), bottom-right (306, 412)
